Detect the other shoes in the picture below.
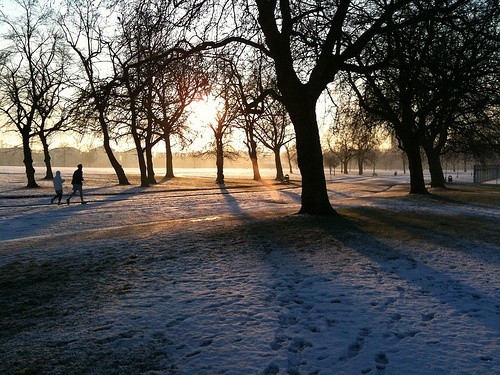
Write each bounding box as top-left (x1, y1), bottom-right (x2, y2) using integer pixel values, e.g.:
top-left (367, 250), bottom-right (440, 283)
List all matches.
top-left (66, 198), bottom-right (70, 205)
top-left (58, 203), bottom-right (61, 205)
top-left (50, 198), bottom-right (53, 204)
top-left (81, 201), bottom-right (87, 203)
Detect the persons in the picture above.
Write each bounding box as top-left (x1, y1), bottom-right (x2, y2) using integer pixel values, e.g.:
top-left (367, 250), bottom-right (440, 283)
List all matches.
top-left (66, 164), bottom-right (87, 205)
top-left (51, 171), bottom-right (65, 205)
